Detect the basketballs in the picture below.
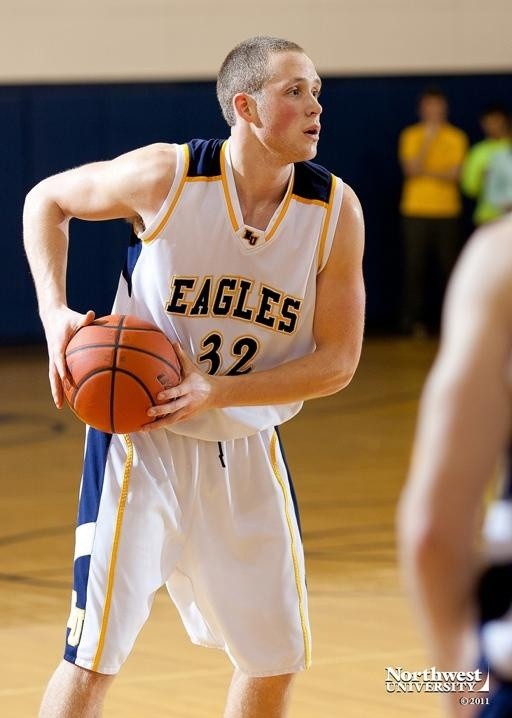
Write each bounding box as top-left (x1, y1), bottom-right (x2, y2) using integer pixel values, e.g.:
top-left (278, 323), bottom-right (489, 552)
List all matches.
top-left (61, 315), bottom-right (181, 433)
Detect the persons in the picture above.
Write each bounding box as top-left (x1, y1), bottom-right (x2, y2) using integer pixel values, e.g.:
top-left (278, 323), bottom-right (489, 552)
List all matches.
top-left (20, 33), bottom-right (370, 717)
top-left (385, 93), bottom-right (469, 343)
top-left (393, 203), bottom-right (512, 718)
top-left (456, 104), bottom-right (510, 230)
